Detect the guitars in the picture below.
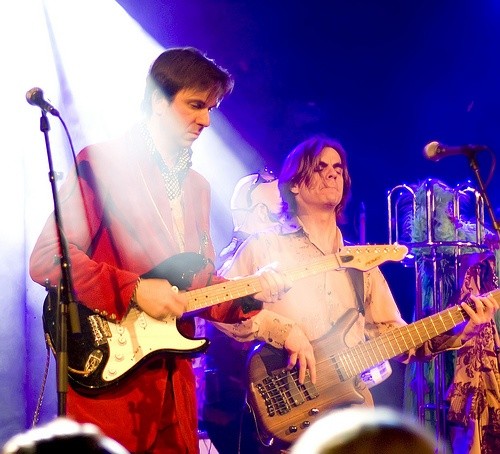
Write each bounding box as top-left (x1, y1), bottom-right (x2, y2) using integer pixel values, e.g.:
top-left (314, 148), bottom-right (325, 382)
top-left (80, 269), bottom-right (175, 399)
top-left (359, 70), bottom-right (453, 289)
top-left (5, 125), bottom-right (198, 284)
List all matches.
top-left (243, 287), bottom-right (499, 448)
top-left (42, 242), bottom-right (409, 398)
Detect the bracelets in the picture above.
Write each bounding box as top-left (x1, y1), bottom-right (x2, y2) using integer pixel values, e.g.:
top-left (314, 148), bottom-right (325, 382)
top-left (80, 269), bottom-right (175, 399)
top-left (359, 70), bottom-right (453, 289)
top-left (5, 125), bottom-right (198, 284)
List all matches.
top-left (130, 277), bottom-right (142, 305)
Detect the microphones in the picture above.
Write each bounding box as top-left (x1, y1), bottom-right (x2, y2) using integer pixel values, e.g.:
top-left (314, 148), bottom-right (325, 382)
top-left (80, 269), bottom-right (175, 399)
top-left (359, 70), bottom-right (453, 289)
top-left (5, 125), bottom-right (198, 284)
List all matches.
top-left (423, 141), bottom-right (485, 161)
top-left (26, 87), bottom-right (60, 117)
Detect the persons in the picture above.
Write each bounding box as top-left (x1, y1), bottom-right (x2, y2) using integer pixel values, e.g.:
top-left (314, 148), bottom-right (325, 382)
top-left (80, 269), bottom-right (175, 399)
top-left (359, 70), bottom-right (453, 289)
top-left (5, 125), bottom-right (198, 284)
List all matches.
top-left (288, 406), bottom-right (443, 454)
top-left (29, 47), bottom-right (294, 454)
top-left (193, 174), bottom-right (284, 454)
top-left (224, 137), bottom-right (499, 454)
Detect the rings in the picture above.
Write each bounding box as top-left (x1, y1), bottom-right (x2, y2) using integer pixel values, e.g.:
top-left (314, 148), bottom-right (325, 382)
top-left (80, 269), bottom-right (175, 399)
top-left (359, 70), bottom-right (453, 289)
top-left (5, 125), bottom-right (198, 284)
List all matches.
top-left (272, 291), bottom-right (279, 296)
